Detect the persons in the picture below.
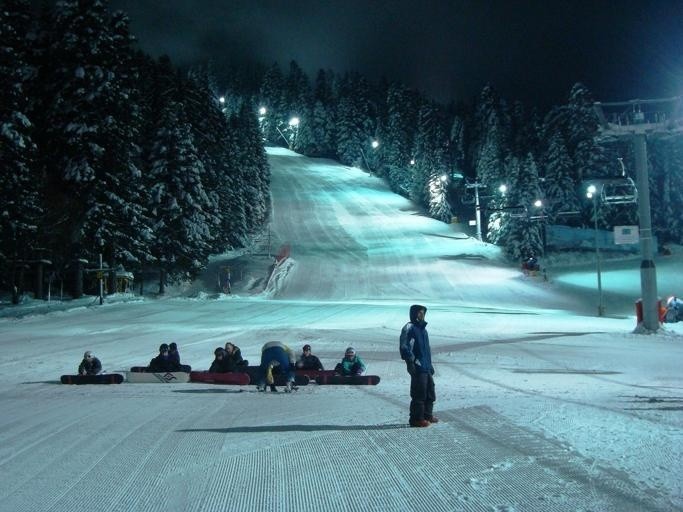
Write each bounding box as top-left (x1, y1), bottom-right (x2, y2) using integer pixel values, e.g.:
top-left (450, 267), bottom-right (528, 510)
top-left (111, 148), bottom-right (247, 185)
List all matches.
top-left (295, 345), bottom-right (324, 371)
top-left (527, 257), bottom-right (537, 270)
top-left (400, 306), bottom-right (439, 426)
top-left (168, 343), bottom-right (180, 363)
top-left (257, 341), bottom-right (296, 392)
top-left (149, 344), bottom-right (179, 372)
top-left (663, 305), bottom-right (679, 323)
top-left (208, 347), bottom-right (239, 373)
top-left (80, 352), bottom-right (101, 375)
top-left (224, 269), bottom-right (231, 292)
top-left (335, 349), bottom-right (366, 377)
top-left (225, 343), bottom-right (248, 365)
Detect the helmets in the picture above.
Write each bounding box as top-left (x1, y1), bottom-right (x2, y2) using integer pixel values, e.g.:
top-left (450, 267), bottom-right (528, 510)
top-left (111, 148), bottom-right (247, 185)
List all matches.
top-left (302, 344), bottom-right (310, 351)
top-left (344, 348), bottom-right (355, 356)
top-left (159, 344), bottom-right (169, 353)
top-left (84, 351), bottom-right (93, 361)
top-left (214, 347), bottom-right (224, 355)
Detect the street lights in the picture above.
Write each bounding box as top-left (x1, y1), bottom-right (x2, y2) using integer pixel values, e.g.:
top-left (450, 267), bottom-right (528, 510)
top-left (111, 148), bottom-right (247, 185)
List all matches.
top-left (585, 186), bottom-right (606, 317)
top-left (532, 198), bottom-right (546, 283)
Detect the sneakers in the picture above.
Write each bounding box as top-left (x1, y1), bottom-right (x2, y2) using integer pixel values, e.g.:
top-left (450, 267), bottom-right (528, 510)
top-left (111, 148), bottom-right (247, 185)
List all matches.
top-left (284, 382), bottom-right (292, 392)
top-left (258, 383), bottom-right (265, 392)
top-left (428, 416), bottom-right (438, 423)
top-left (408, 418), bottom-right (430, 427)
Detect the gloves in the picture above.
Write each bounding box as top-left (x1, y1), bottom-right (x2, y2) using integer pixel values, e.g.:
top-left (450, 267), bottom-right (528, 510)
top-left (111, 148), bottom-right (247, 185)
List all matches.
top-left (269, 383), bottom-right (277, 392)
